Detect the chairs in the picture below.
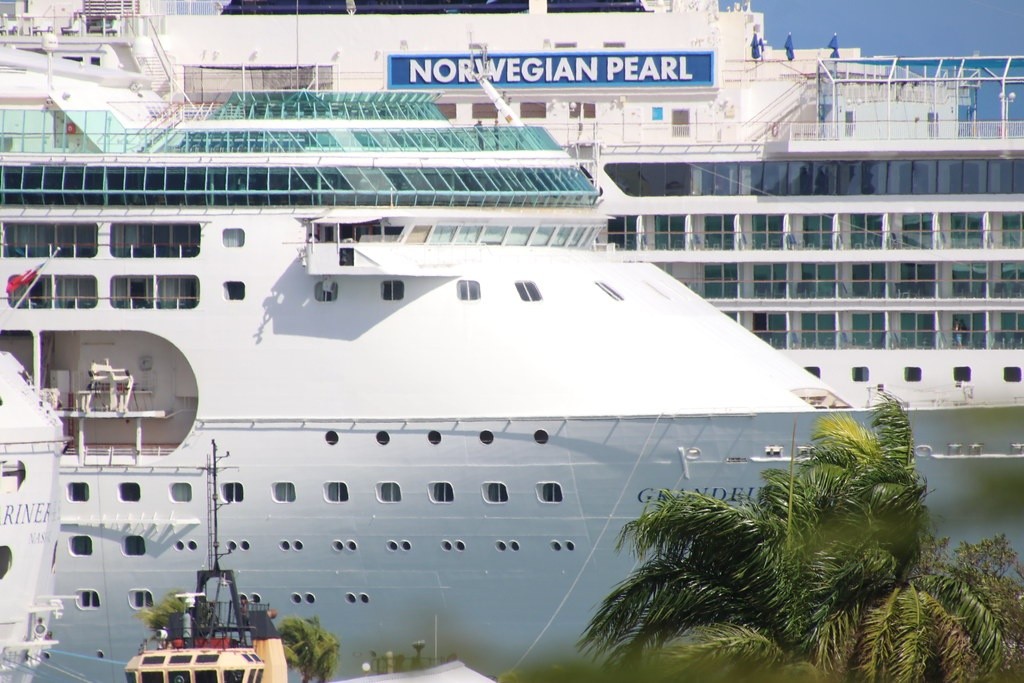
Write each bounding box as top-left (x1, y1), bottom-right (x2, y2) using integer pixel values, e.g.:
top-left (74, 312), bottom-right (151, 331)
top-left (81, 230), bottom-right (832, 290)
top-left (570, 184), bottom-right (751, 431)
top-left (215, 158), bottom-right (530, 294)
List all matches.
top-left (893, 333), bottom-right (909, 350)
top-left (796, 283), bottom-right (808, 298)
top-left (891, 233), bottom-right (903, 249)
top-left (175, 138), bottom-right (305, 153)
top-left (774, 283), bottom-right (786, 298)
top-left (1010, 283), bottom-right (1022, 298)
top-left (755, 283), bottom-right (769, 298)
top-left (841, 282), bottom-right (855, 298)
top-left (101, 20), bottom-right (118, 37)
top-left (61, 20), bottom-right (80, 36)
top-left (994, 282), bottom-right (1007, 297)
top-left (32, 24), bottom-right (53, 37)
top-left (1010, 333), bottom-right (1023, 349)
top-left (909, 284), bottom-right (921, 297)
top-left (842, 333), bottom-right (857, 348)
top-left (791, 333), bottom-right (806, 348)
top-left (0, 25), bottom-right (18, 36)
top-left (895, 283), bottom-right (909, 298)
top-left (993, 333), bottom-right (1006, 349)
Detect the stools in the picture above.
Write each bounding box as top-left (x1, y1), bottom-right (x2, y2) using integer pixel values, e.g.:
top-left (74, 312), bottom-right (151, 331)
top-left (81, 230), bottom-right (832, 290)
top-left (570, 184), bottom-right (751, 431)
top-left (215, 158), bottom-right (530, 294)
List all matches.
top-left (808, 243), bottom-right (815, 249)
top-left (713, 243), bottom-right (720, 250)
top-left (855, 243), bottom-right (862, 250)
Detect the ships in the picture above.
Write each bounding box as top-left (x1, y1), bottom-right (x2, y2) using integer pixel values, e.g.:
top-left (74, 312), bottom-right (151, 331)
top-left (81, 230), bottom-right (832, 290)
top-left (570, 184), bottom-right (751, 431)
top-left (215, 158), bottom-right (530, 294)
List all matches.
top-left (0, 0), bottom-right (1024, 683)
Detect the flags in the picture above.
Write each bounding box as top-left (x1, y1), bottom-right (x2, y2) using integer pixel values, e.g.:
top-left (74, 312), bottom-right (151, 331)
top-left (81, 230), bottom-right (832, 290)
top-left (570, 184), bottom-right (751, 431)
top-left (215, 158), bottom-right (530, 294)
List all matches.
top-left (5, 262), bottom-right (45, 294)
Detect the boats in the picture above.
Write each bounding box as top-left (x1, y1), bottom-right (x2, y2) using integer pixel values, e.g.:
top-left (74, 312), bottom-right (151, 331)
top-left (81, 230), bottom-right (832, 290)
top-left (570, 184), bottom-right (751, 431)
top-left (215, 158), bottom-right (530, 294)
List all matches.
top-left (125, 438), bottom-right (288, 683)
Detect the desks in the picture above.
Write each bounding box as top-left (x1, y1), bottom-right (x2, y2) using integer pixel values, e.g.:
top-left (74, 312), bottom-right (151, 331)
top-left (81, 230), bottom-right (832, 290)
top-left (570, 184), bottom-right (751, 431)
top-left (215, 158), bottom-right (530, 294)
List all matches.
top-left (57, 409), bottom-right (172, 464)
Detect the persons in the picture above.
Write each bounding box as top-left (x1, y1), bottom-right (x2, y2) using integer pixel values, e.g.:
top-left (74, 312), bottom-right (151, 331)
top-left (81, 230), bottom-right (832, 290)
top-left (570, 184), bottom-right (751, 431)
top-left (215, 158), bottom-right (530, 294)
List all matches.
top-left (474, 119), bottom-right (485, 151)
top-left (309, 232), bottom-right (319, 243)
top-left (493, 120), bottom-right (500, 150)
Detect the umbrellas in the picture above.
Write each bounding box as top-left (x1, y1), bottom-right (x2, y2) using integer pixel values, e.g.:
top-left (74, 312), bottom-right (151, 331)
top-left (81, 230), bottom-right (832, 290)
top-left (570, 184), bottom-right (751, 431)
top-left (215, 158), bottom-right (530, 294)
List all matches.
top-left (827, 36), bottom-right (839, 58)
top-left (784, 36), bottom-right (795, 59)
top-left (750, 33), bottom-right (765, 59)
